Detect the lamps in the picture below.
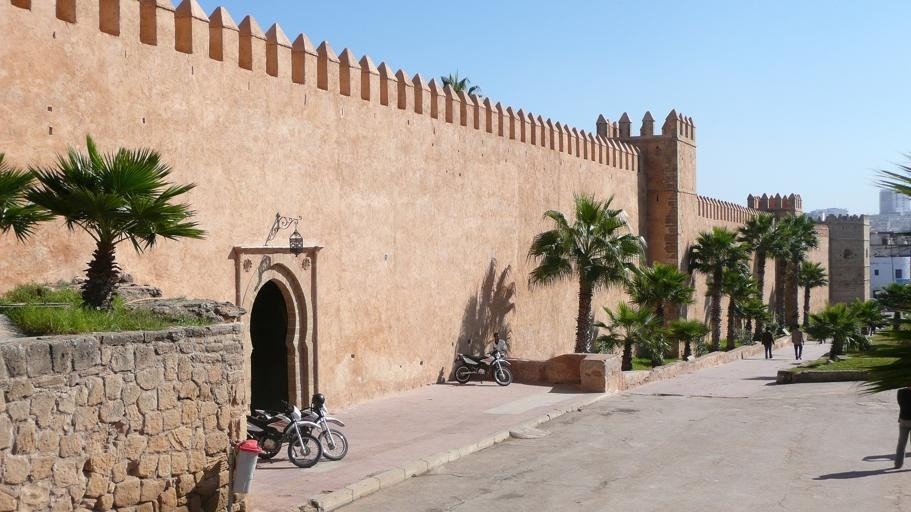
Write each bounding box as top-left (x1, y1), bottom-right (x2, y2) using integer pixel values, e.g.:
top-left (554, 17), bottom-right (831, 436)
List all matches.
top-left (278, 214), bottom-right (305, 257)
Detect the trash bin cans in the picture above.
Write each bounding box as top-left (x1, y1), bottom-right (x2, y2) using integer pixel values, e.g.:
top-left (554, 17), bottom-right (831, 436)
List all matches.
top-left (861, 326), bottom-right (869, 335)
top-left (233, 439), bottom-right (262, 494)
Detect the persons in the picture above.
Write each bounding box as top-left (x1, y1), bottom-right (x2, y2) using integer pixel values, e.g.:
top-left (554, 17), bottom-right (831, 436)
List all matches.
top-left (895, 386), bottom-right (911, 469)
top-left (762, 326), bottom-right (775, 359)
top-left (486, 331), bottom-right (508, 380)
top-left (791, 322), bottom-right (804, 360)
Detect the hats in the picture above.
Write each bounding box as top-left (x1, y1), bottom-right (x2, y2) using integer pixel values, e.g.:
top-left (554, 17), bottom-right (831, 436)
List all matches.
top-left (765, 327), bottom-right (771, 330)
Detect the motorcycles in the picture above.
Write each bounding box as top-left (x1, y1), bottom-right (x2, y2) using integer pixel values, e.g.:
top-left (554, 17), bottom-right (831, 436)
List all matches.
top-left (454, 346), bottom-right (513, 386)
top-left (247, 394), bottom-right (348, 468)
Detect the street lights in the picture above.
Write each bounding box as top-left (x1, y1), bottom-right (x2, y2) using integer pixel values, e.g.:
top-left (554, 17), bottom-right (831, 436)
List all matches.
top-left (882, 236), bottom-right (894, 285)
